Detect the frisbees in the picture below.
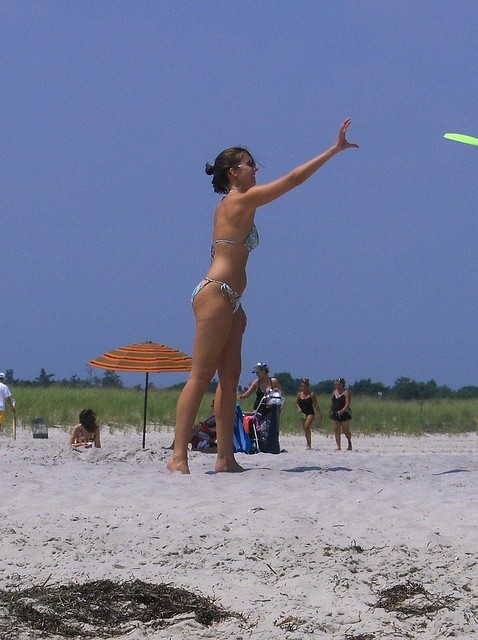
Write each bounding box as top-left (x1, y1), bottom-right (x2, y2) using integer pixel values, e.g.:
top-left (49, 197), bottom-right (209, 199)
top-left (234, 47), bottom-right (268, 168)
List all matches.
top-left (443, 133), bottom-right (478, 146)
top-left (301, 413), bottom-right (307, 419)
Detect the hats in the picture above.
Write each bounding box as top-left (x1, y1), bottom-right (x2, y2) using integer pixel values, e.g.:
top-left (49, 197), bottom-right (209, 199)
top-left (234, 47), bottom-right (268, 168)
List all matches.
top-left (300, 378), bottom-right (309, 384)
top-left (336, 378), bottom-right (345, 383)
top-left (0, 373), bottom-right (6, 379)
top-left (252, 362), bottom-right (269, 373)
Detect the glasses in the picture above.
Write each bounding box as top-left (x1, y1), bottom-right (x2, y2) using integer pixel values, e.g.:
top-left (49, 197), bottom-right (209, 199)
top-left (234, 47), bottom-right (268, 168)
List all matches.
top-left (235, 159), bottom-right (256, 169)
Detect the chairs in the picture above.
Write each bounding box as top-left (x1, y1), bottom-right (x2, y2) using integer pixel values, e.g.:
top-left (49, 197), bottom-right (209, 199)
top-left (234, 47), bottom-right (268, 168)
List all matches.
top-left (242, 397), bottom-right (270, 453)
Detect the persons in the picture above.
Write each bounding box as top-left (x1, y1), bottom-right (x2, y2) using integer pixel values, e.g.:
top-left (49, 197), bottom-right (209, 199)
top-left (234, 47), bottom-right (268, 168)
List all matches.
top-left (236, 362), bottom-right (282, 424)
top-left (69, 409), bottom-right (101, 451)
top-left (295, 378), bottom-right (321, 449)
top-left (207, 432), bottom-right (217, 448)
top-left (329, 378), bottom-right (353, 451)
top-left (167, 117), bottom-right (359, 476)
top-left (0, 372), bottom-right (15, 435)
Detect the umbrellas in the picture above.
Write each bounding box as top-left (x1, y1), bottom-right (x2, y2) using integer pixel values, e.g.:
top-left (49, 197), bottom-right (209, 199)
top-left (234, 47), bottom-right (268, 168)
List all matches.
top-left (88, 340), bottom-right (193, 449)
